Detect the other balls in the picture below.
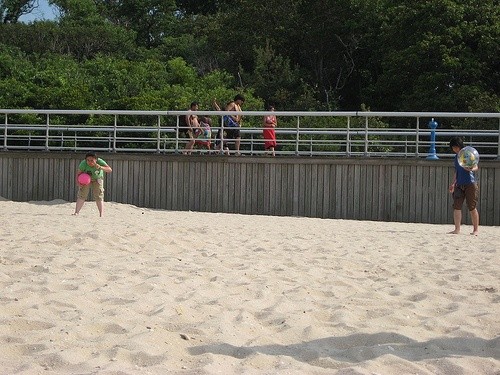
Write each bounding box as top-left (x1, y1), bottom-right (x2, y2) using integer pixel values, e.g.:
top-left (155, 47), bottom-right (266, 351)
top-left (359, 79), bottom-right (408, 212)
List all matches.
top-left (78, 172), bottom-right (91, 186)
top-left (457, 146), bottom-right (479, 169)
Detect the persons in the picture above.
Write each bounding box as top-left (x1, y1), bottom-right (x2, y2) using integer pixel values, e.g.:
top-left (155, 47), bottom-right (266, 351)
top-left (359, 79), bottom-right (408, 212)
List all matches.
top-left (262, 105), bottom-right (278, 157)
top-left (71, 151), bottom-right (112, 217)
top-left (214, 94), bottom-right (246, 156)
top-left (446, 136), bottom-right (479, 236)
top-left (196, 109), bottom-right (213, 156)
top-left (181, 101), bottom-right (200, 156)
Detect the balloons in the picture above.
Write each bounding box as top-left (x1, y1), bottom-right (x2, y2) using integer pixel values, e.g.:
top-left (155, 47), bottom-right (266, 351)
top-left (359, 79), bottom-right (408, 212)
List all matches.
top-left (456, 146), bottom-right (480, 169)
